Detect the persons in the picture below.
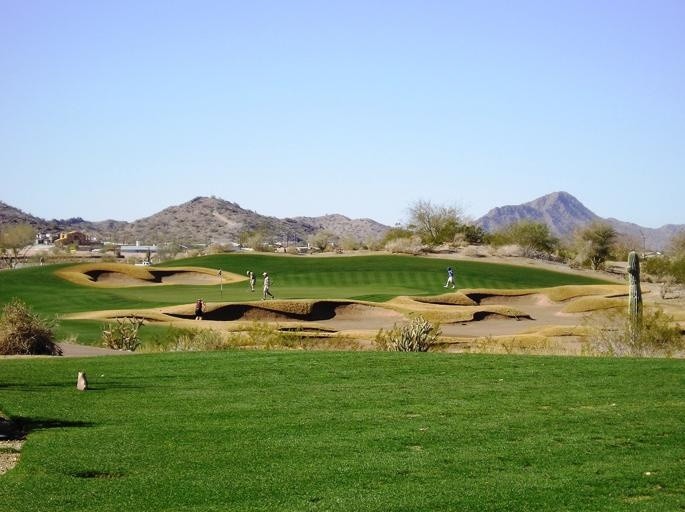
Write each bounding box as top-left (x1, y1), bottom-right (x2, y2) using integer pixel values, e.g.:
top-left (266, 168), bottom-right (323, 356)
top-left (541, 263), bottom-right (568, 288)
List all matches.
top-left (442, 267), bottom-right (456, 289)
top-left (247, 271), bottom-right (255, 292)
top-left (261, 272), bottom-right (274, 301)
top-left (195, 298), bottom-right (203, 321)
top-left (40, 256), bottom-right (44, 267)
